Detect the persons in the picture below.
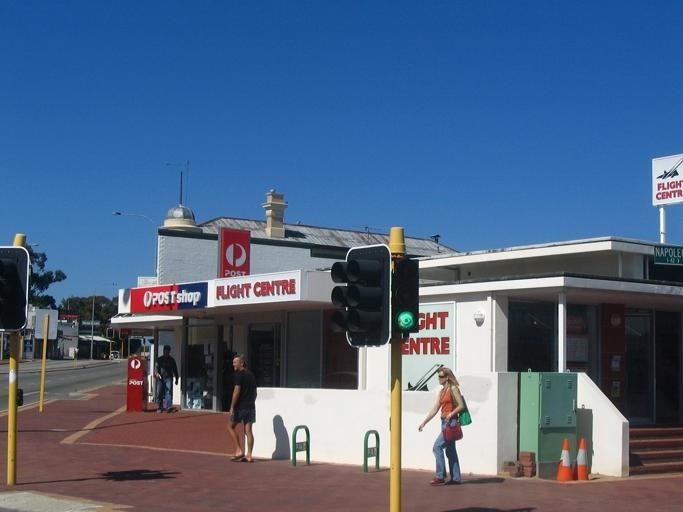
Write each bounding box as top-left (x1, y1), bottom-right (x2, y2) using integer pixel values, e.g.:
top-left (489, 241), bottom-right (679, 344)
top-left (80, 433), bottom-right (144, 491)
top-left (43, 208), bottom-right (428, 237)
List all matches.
top-left (153, 345), bottom-right (179, 414)
top-left (226, 355), bottom-right (257, 463)
top-left (417, 368), bottom-right (464, 485)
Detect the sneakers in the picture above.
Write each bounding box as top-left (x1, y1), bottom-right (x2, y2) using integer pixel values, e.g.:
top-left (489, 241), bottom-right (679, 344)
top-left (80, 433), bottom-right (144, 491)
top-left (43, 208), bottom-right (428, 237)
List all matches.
top-left (167, 406), bottom-right (176, 413)
top-left (429, 477), bottom-right (445, 486)
top-left (157, 405), bottom-right (163, 413)
top-left (444, 479), bottom-right (461, 485)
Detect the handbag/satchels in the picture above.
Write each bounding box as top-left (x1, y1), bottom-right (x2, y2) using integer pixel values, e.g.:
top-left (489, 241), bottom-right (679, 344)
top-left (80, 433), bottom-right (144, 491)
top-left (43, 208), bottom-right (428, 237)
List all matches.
top-left (444, 423), bottom-right (463, 442)
top-left (459, 395), bottom-right (472, 426)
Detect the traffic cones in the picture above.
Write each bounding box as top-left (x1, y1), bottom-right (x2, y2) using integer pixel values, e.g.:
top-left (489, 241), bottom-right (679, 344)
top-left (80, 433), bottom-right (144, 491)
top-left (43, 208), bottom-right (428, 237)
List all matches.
top-left (555, 437), bottom-right (573, 482)
top-left (573, 436), bottom-right (590, 480)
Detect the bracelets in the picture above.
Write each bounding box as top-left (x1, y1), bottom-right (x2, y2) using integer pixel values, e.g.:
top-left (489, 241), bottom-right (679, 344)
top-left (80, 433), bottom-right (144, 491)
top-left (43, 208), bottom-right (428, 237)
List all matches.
top-left (449, 413), bottom-right (452, 418)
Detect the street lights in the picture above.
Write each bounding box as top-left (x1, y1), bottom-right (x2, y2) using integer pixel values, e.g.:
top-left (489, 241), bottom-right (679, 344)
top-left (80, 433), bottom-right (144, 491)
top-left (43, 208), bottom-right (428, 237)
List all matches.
top-left (111, 212), bottom-right (156, 277)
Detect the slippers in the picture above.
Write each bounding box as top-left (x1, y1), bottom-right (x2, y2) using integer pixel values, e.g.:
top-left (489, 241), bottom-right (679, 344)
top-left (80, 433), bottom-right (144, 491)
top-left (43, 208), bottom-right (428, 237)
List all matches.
top-left (230, 453), bottom-right (254, 462)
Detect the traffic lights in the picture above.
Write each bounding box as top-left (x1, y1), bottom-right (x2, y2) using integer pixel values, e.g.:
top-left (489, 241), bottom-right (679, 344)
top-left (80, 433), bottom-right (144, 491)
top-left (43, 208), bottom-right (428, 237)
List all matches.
top-left (394, 257), bottom-right (418, 332)
